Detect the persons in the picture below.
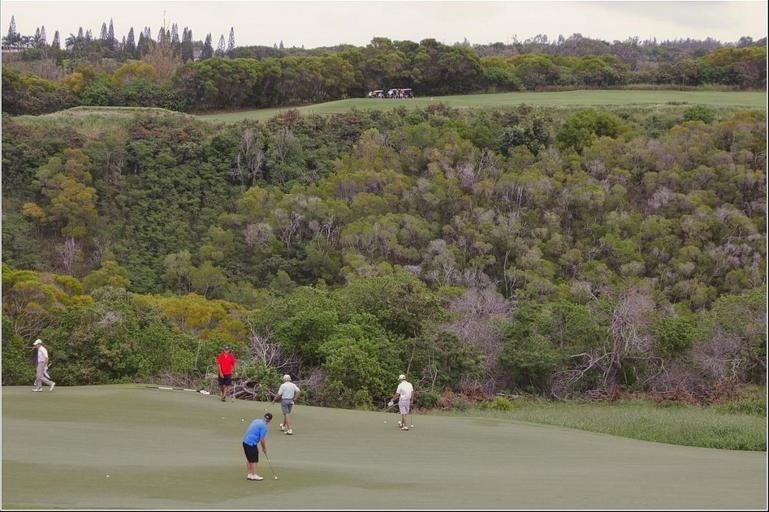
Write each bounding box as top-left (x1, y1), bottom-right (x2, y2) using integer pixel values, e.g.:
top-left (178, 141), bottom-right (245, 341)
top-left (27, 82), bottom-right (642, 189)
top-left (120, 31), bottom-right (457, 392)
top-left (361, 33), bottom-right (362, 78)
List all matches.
top-left (242, 412), bottom-right (272, 481)
top-left (271, 374), bottom-right (301, 434)
top-left (32, 339), bottom-right (56, 392)
top-left (216, 346), bottom-right (236, 401)
top-left (391, 374), bottom-right (414, 431)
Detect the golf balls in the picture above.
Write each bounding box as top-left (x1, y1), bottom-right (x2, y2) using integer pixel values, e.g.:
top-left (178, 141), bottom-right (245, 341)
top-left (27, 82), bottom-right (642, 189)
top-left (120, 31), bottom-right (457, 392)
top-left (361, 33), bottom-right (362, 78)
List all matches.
top-left (241, 419), bottom-right (244, 421)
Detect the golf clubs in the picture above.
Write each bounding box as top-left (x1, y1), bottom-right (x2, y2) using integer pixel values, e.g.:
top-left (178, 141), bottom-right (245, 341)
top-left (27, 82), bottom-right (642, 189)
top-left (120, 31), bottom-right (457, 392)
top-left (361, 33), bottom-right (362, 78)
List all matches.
top-left (264, 455), bottom-right (278, 480)
top-left (410, 408), bottom-right (413, 428)
top-left (264, 401), bottom-right (273, 410)
top-left (384, 398), bottom-right (387, 423)
top-left (232, 376), bottom-right (235, 401)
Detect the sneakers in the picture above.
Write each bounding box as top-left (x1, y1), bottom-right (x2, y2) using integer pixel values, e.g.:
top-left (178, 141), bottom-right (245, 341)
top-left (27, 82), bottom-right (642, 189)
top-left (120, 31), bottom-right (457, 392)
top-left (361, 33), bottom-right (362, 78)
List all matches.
top-left (48, 383), bottom-right (56, 392)
top-left (280, 424), bottom-right (293, 434)
top-left (398, 421), bottom-right (408, 430)
top-left (32, 388), bottom-right (43, 392)
top-left (247, 473), bottom-right (263, 480)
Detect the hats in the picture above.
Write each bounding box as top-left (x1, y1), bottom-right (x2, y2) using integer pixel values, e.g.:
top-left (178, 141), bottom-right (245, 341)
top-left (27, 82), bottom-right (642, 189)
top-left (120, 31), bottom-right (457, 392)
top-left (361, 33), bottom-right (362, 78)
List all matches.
top-left (33, 339), bottom-right (42, 345)
top-left (398, 375), bottom-right (406, 380)
